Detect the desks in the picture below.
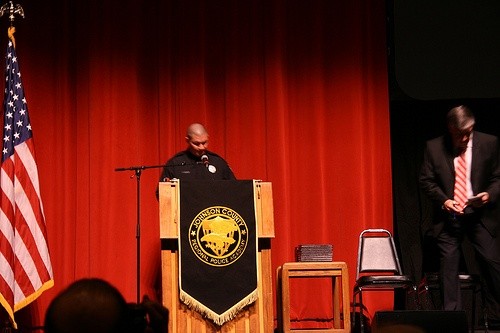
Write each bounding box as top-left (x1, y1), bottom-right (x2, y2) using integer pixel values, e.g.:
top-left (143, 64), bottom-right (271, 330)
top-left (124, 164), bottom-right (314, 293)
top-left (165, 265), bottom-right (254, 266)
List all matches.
top-left (276, 262), bottom-right (350, 333)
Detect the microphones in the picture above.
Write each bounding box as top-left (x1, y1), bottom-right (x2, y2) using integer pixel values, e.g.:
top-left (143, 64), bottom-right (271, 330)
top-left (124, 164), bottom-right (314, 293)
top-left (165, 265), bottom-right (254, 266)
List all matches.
top-left (201, 154), bottom-right (209, 167)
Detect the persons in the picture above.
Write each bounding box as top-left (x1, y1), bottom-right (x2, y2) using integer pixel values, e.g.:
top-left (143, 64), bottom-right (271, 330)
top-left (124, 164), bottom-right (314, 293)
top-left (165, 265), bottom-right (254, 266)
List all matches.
top-left (156, 123), bottom-right (237, 201)
top-left (419, 105), bottom-right (500, 331)
top-left (44, 278), bottom-right (169, 333)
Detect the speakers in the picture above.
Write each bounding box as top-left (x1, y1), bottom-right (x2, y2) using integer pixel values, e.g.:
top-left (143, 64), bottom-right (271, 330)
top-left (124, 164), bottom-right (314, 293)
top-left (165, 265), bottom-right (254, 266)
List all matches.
top-left (370, 310), bottom-right (469, 333)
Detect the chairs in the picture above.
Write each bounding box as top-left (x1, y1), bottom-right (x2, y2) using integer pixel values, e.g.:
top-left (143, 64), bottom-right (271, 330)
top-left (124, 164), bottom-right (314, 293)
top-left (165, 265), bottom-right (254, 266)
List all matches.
top-left (422, 226), bottom-right (479, 333)
top-left (353, 229), bottom-right (420, 333)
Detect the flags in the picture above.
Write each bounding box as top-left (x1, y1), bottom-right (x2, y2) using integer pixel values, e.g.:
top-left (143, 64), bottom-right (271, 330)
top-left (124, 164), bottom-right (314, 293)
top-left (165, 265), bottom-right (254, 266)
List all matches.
top-left (0, 26), bottom-right (55, 333)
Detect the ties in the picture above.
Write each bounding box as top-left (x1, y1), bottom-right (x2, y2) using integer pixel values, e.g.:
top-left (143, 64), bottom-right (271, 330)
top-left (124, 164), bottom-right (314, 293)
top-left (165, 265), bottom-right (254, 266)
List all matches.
top-left (453, 153), bottom-right (469, 212)
